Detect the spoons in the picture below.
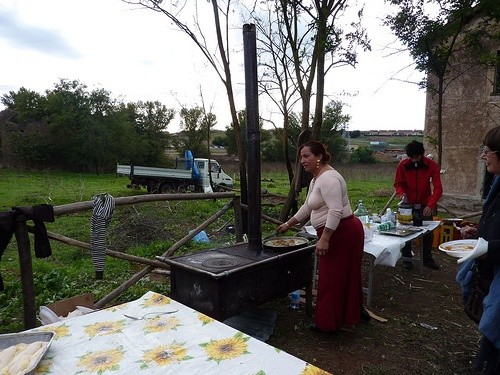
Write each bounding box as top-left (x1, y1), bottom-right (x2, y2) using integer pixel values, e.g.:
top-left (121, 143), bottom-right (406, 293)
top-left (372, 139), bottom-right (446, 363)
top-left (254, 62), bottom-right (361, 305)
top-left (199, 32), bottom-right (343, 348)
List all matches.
top-left (123, 309), bottom-right (177, 319)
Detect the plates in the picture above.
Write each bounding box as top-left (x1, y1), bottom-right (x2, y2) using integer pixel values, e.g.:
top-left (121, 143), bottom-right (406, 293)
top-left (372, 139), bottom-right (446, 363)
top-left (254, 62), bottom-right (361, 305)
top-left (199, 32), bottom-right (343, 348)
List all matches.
top-left (438, 239), bottom-right (478, 257)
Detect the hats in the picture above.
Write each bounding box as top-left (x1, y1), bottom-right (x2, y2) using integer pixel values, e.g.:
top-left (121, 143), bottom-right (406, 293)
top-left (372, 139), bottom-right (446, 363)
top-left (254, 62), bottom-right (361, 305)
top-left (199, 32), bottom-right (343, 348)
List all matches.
top-left (406, 140), bottom-right (425, 158)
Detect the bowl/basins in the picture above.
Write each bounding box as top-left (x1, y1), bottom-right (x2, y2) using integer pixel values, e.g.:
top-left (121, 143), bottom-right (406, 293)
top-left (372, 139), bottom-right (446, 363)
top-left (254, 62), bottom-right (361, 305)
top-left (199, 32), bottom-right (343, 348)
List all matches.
top-left (39, 306), bottom-right (60, 323)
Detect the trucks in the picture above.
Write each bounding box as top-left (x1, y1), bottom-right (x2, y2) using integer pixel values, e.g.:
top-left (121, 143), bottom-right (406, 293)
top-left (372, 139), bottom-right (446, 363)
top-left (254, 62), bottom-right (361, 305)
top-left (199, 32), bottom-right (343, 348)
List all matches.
top-left (116, 150), bottom-right (235, 194)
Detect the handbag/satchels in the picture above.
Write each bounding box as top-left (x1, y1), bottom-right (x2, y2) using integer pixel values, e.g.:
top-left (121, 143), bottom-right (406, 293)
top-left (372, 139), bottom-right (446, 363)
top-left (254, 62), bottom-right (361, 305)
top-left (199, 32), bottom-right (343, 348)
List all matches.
top-left (464, 287), bottom-right (487, 325)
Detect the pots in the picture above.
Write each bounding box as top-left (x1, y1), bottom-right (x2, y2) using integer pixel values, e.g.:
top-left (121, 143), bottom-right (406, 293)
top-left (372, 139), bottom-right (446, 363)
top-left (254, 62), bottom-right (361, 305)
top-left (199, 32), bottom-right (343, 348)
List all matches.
top-left (262, 236), bottom-right (308, 248)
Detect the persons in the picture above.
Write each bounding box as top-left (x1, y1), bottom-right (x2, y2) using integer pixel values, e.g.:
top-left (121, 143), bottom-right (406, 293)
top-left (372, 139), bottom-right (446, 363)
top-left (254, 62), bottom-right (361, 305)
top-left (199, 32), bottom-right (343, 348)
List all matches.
top-left (456, 125), bottom-right (500, 375)
top-left (276, 141), bottom-right (365, 335)
top-left (394, 140), bottom-right (442, 270)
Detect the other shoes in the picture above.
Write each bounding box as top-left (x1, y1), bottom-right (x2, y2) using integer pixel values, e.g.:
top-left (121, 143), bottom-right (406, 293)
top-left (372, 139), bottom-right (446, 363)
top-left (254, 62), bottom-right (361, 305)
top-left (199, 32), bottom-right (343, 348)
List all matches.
top-left (340, 324), bottom-right (356, 333)
top-left (403, 260), bottom-right (413, 270)
top-left (309, 323), bottom-right (338, 335)
top-left (423, 258), bottom-right (440, 269)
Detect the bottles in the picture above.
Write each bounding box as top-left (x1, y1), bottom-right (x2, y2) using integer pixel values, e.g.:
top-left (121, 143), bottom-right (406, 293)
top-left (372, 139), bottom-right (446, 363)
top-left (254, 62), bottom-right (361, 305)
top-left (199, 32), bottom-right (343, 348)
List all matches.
top-left (290, 289), bottom-right (301, 309)
top-left (353, 191), bottom-right (423, 243)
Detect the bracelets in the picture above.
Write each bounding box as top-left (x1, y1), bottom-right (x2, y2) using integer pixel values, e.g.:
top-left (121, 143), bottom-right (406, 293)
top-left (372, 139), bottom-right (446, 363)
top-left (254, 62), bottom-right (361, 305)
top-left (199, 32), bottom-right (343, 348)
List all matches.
top-left (285, 222), bottom-right (291, 229)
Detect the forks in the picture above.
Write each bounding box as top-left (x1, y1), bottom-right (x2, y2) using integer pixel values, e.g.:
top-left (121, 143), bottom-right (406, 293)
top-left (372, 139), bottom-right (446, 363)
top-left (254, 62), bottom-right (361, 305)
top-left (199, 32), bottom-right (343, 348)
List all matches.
top-left (454, 223), bottom-right (476, 237)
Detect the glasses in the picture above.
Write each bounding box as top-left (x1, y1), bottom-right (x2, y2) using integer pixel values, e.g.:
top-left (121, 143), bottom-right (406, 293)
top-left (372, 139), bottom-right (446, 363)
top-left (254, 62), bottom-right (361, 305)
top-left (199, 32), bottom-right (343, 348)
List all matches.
top-left (480, 148), bottom-right (498, 156)
